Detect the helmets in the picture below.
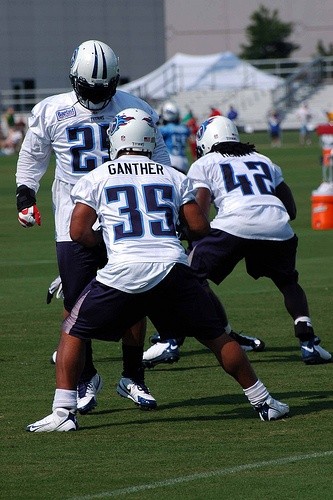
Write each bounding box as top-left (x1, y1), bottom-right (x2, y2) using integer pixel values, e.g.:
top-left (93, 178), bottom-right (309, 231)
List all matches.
top-left (106, 107), bottom-right (158, 161)
top-left (68, 39), bottom-right (120, 112)
top-left (160, 102), bottom-right (179, 122)
top-left (194, 115), bottom-right (241, 158)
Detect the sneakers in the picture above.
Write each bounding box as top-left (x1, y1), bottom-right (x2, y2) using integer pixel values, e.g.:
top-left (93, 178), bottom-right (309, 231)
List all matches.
top-left (301, 335), bottom-right (333, 364)
top-left (115, 371), bottom-right (158, 410)
top-left (228, 328), bottom-right (266, 352)
top-left (25, 406), bottom-right (79, 432)
top-left (75, 372), bottom-right (104, 415)
top-left (252, 394), bottom-right (291, 420)
top-left (142, 332), bottom-right (181, 369)
top-left (50, 349), bottom-right (58, 364)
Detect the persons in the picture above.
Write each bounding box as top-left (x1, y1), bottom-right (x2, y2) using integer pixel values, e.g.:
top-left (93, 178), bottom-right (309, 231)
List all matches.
top-left (24, 108), bottom-right (292, 433)
top-left (15, 37), bottom-right (172, 412)
top-left (317, 112), bottom-right (333, 182)
top-left (0, 106), bottom-right (25, 156)
top-left (269, 108), bottom-right (282, 148)
top-left (302, 102), bottom-right (313, 144)
top-left (139, 116), bottom-right (333, 369)
top-left (156, 102), bottom-right (238, 174)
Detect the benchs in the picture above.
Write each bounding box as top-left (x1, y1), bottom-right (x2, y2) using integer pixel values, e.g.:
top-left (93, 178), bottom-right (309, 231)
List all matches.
top-left (153, 83), bottom-right (333, 131)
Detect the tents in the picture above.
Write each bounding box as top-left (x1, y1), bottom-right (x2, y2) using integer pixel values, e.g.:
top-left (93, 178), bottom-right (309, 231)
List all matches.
top-left (116, 52), bottom-right (333, 132)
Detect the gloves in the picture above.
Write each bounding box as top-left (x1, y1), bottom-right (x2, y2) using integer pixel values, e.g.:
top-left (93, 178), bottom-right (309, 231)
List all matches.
top-left (46, 274), bottom-right (64, 304)
top-left (16, 185), bottom-right (42, 228)
